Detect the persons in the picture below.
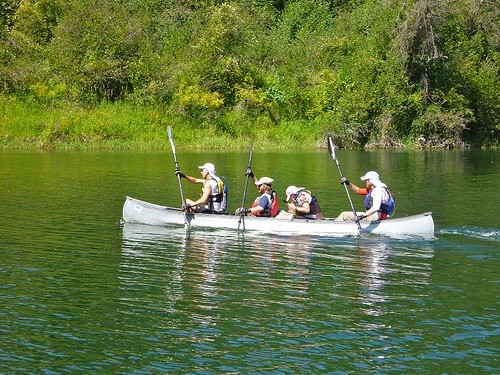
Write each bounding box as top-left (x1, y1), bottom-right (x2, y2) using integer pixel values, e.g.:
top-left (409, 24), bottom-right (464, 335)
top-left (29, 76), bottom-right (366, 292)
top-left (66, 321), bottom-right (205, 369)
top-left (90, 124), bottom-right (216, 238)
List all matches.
top-left (334, 170), bottom-right (395, 223)
top-left (175, 163), bottom-right (227, 214)
top-left (235, 169), bottom-right (279, 217)
top-left (282, 185), bottom-right (322, 220)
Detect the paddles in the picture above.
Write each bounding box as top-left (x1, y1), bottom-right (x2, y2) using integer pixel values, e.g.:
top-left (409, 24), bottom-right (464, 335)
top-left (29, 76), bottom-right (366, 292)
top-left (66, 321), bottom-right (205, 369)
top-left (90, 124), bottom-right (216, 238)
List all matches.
top-left (328, 135), bottom-right (364, 238)
top-left (167, 125), bottom-right (190, 227)
top-left (236, 148), bottom-right (253, 232)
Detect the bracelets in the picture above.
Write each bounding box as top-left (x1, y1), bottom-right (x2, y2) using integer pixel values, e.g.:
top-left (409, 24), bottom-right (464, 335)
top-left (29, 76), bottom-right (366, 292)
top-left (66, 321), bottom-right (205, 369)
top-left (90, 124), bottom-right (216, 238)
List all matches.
top-left (296, 206), bottom-right (297, 210)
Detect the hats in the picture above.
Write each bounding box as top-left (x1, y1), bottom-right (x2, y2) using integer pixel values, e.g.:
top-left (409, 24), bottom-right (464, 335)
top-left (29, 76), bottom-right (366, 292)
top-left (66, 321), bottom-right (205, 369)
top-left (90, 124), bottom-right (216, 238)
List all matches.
top-left (255, 176), bottom-right (274, 186)
top-left (285, 185), bottom-right (300, 201)
top-left (197, 163), bottom-right (217, 175)
top-left (360, 171), bottom-right (380, 182)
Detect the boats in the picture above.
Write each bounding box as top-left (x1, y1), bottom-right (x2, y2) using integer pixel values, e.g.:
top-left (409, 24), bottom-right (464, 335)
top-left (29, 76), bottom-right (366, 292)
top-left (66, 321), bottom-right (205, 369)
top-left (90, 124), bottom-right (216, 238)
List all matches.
top-left (122, 195), bottom-right (440, 241)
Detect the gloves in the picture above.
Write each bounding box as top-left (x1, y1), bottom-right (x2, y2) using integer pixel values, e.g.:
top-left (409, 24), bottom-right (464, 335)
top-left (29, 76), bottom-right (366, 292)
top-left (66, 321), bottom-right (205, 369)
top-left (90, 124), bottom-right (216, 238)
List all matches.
top-left (244, 168), bottom-right (254, 178)
top-left (354, 215), bottom-right (365, 223)
top-left (181, 203), bottom-right (191, 210)
top-left (235, 208), bottom-right (248, 217)
top-left (175, 170), bottom-right (186, 181)
top-left (340, 176), bottom-right (350, 185)
